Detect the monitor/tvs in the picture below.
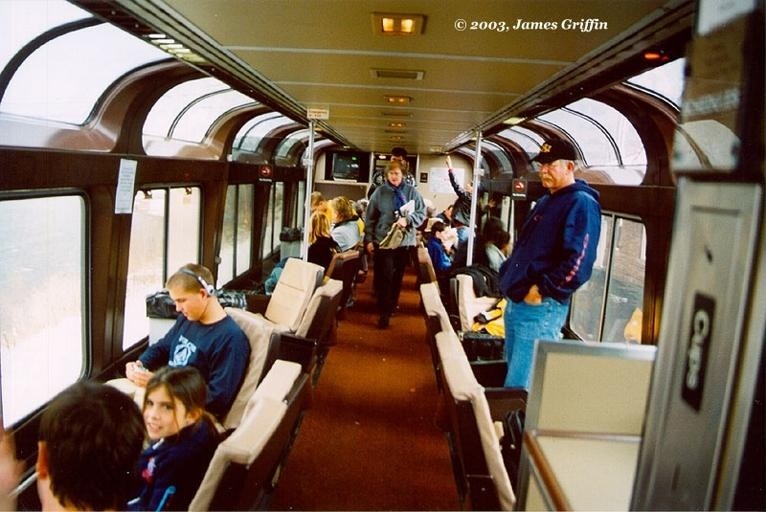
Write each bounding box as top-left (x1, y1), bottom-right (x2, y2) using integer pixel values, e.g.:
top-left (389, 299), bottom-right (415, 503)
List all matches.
top-left (331, 153), bottom-right (361, 183)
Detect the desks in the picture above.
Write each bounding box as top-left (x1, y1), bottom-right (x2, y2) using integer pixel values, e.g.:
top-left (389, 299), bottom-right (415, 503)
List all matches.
top-left (522, 429), bottom-right (642, 512)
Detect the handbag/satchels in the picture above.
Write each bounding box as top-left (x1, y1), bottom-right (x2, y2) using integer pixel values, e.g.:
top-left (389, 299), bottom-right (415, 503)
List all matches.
top-left (379, 223), bottom-right (404, 251)
top-left (146, 290), bottom-right (177, 320)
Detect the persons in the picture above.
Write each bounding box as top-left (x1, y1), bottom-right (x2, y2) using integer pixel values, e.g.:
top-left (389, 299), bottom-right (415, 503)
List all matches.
top-left (0, 380), bottom-right (148, 512)
top-left (126, 364), bottom-right (237, 512)
top-left (303, 147), bottom-right (511, 329)
top-left (498, 138), bottom-right (602, 388)
top-left (125, 263), bottom-right (252, 410)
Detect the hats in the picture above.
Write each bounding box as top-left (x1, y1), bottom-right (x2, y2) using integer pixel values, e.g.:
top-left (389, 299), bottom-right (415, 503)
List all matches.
top-left (529, 139), bottom-right (577, 163)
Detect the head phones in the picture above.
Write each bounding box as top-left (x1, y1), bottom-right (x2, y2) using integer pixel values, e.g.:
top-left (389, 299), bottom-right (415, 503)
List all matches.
top-left (179, 267), bottom-right (215, 296)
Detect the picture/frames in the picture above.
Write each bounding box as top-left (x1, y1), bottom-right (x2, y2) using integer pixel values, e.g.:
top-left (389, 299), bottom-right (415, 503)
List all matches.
top-left (516, 339), bottom-right (657, 512)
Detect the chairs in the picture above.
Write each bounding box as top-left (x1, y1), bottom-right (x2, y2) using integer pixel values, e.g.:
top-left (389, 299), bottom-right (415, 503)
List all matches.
top-left (188, 228), bottom-right (365, 512)
top-left (420, 282), bottom-right (505, 393)
top-left (422, 218), bottom-right (444, 247)
top-left (448, 386), bottom-right (528, 511)
top-left (417, 247), bottom-right (442, 295)
top-left (434, 331), bottom-right (528, 361)
top-left (435, 362), bottom-right (508, 394)
top-left (456, 273), bottom-right (509, 335)
top-left (419, 241), bottom-right (424, 248)
top-left (450, 278), bottom-right (461, 330)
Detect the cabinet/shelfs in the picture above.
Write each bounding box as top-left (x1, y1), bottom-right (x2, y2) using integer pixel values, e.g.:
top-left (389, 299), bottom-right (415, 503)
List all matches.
top-left (315, 180), bottom-right (371, 202)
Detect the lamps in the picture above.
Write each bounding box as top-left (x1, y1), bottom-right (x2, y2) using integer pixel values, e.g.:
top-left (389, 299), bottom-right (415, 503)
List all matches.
top-left (623, 306), bottom-right (644, 345)
